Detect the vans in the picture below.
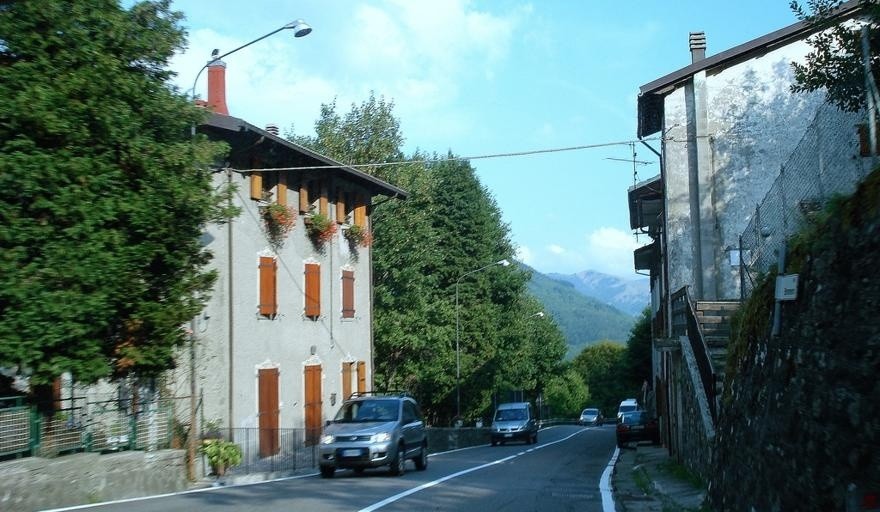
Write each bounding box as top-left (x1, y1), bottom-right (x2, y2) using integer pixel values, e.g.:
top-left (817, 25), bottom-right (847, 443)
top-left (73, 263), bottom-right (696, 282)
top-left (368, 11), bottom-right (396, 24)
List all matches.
top-left (488, 401), bottom-right (538, 445)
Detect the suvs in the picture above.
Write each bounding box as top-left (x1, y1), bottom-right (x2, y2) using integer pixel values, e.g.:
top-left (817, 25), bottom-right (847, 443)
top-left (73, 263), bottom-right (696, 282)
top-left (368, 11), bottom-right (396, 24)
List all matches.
top-left (318, 390), bottom-right (431, 478)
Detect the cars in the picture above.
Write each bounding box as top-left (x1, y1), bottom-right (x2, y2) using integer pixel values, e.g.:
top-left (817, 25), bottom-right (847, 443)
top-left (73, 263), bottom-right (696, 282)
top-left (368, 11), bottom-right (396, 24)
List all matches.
top-left (616, 400), bottom-right (639, 418)
top-left (577, 408), bottom-right (604, 427)
top-left (616, 409), bottom-right (661, 451)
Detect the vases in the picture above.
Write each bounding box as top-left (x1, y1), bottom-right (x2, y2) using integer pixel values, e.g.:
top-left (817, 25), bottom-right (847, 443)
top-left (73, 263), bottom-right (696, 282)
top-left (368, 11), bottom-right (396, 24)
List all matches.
top-left (304, 209), bottom-right (340, 259)
top-left (344, 224), bottom-right (376, 265)
top-left (259, 203), bottom-right (299, 253)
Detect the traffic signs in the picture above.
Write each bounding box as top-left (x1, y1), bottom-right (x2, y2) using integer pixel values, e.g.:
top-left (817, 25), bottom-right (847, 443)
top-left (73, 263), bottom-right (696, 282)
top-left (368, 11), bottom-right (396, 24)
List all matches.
top-left (515, 312), bottom-right (544, 329)
top-left (455, 260), bottom-right (511, 432)
top-left (185, 17), bottom-right (312, 484)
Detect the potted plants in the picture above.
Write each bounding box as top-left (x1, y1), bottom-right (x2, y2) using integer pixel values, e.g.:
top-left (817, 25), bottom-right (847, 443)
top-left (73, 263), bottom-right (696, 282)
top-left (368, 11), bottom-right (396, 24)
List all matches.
top-left (200, 439), bottom-right (242, 475)
